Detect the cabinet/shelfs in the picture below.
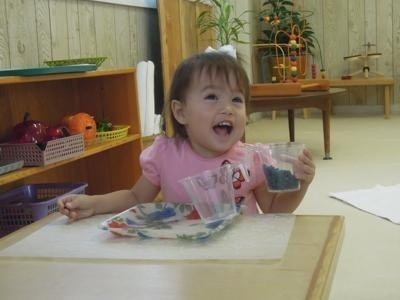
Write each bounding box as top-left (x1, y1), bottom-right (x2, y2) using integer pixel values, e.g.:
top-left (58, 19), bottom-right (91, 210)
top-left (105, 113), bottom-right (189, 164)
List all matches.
top-left (0, 66), bottom-right (143, 240)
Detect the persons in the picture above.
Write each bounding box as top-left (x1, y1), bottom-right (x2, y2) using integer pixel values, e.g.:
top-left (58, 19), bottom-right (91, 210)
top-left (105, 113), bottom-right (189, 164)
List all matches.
top-left (57, 52), bottom-right (316, 223)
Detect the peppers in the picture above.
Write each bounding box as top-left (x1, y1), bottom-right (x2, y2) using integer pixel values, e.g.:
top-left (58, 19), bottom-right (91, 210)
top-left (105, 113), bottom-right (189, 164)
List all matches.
top-left (98, 120), bottom-right (113, 133)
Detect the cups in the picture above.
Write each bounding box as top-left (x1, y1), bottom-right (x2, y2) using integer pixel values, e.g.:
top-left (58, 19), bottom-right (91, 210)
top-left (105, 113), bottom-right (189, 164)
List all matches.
top-left (176, 163), bottom-right (239, 225)
top-left (254, 141), bottom-right (307, 195)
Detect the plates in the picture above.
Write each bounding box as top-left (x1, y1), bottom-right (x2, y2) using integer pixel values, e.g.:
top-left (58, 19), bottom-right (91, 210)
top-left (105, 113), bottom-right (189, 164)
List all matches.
top-left (98, 200), bottom-right (249, 242)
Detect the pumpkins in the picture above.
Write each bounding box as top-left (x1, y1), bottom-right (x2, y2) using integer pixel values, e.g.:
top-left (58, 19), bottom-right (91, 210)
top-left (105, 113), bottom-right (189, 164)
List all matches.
top-left (8, 111), bottom-right (97, 151)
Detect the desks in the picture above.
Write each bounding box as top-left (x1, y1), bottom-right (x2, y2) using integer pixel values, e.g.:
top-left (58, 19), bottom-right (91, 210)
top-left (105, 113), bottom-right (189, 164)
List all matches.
top-left (0, 211), bottom-right (345, 300)
top-left (271, 77), bottom-right (396, 119)
top-left (241, 89), bottom-right (333, 161)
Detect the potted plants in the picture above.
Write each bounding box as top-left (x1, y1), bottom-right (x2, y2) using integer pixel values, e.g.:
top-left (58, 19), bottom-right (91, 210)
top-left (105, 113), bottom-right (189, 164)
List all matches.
top-left (255, 0), bottom-right (324, 82)
